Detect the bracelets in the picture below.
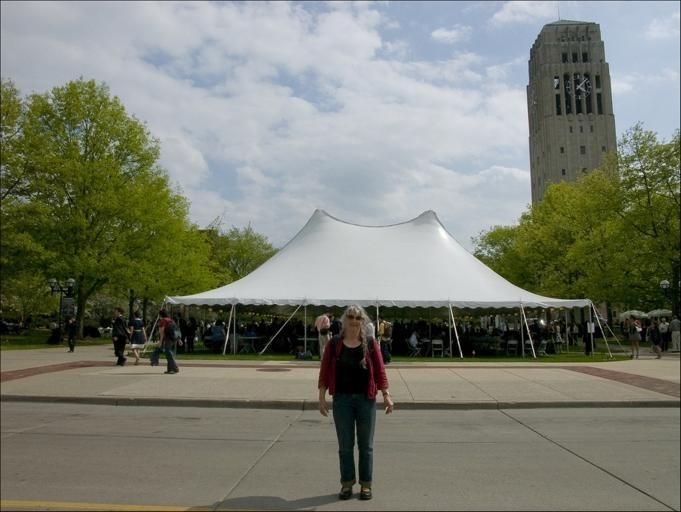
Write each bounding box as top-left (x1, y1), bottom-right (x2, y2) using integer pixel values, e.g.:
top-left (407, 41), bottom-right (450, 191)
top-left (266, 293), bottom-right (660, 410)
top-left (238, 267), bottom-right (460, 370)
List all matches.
top-left (382, 391), bottom-right (390, 396)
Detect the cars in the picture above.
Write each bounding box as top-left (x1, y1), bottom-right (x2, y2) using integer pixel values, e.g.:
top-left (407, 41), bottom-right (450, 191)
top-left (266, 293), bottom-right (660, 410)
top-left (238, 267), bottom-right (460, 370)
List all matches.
top-left (523, 317), bottom-right (546, 328)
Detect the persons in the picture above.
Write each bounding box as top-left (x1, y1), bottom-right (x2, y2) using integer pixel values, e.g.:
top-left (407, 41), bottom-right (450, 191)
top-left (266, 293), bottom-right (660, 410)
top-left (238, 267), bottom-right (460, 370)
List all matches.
top-left (317, 303), bottom-right (395, 500)
top-left (108, 302), bottom-right (681, 375)
top-left (44, 316), bottom-right (79, 354)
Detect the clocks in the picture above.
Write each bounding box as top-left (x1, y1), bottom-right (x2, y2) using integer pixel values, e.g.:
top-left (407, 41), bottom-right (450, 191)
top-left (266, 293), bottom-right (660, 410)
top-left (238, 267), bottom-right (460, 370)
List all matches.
top-left (565, 74), bottom-right (592, 101)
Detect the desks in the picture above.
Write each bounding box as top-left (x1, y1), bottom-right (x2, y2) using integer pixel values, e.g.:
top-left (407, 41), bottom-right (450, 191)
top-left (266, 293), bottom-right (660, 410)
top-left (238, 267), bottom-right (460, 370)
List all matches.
top-left (298, 337), bottom-right (318, 360)
top-left (238, 336), bottom-right (262, 354)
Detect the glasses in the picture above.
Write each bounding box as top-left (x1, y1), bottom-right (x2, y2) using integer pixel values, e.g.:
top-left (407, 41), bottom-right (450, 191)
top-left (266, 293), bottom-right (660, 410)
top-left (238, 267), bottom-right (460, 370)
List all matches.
top-left (346, 314), bottom-right (362, 320)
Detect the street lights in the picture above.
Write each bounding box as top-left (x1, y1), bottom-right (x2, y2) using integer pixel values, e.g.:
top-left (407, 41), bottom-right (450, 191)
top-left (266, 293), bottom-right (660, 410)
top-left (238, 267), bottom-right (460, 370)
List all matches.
top-left (48, 277), bottom-right (76, 345)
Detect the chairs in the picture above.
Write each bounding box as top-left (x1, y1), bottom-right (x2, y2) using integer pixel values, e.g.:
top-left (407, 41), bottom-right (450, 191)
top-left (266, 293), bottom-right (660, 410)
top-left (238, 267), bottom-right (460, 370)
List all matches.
top-left (525, 340), bottom-right (534, 355)
top-left (431, 339), bottom-right (443, 358)
top-left (405, 338), bottom-right (421, 357)
top-left (507, 340), bottom-right (519, 356)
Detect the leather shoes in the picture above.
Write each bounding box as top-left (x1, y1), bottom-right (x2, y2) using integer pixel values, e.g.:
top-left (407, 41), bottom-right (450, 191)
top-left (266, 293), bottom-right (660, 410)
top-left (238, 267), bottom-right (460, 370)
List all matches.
top-left (339, 485), bottom-right (352, 500)
top-left (360, 487), bottom-right (371, 499)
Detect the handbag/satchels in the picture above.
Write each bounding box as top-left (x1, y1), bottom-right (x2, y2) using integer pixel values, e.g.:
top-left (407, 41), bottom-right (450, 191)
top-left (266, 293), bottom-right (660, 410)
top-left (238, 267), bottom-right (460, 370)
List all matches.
top-left (320, 328), bottom-right (327, 336)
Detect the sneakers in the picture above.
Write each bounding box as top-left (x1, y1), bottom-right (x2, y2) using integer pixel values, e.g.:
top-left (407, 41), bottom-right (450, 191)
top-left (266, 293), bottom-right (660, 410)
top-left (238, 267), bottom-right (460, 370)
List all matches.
top-left (164, 368), bottom-right (179, 374)
top-left (117, 357), bottom-right (140, 366)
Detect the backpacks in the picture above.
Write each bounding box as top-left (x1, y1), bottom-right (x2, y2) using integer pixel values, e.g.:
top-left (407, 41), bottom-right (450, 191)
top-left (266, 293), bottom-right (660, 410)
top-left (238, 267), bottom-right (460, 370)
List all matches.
top-left (382, 321), bottom-right (393, 338)
top-left (165, 319), bottom-right (181, 341)
